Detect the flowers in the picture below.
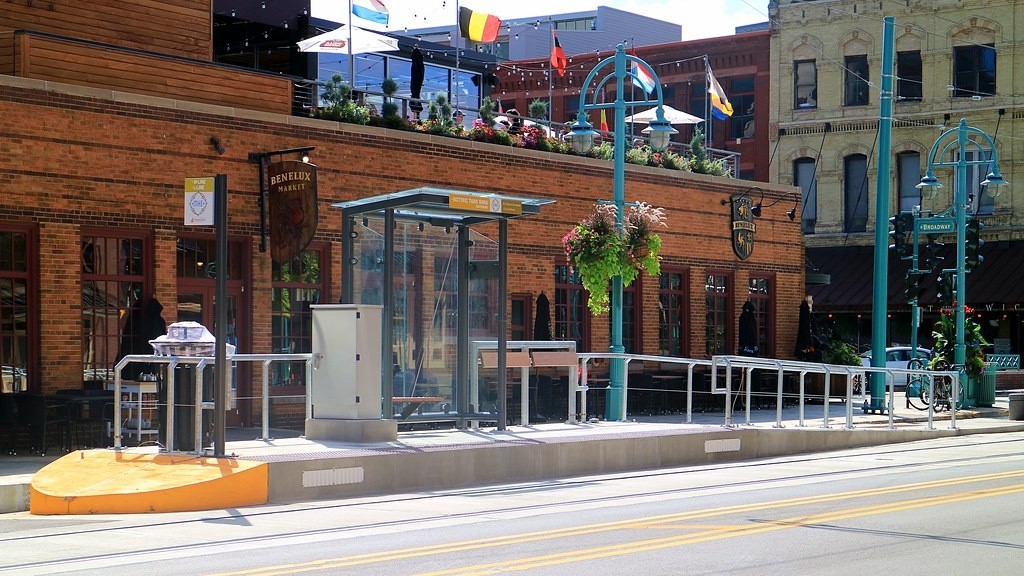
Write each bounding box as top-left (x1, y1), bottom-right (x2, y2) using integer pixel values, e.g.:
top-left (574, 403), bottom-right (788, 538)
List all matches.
top-left (562, 199), bottom-right (669, 315)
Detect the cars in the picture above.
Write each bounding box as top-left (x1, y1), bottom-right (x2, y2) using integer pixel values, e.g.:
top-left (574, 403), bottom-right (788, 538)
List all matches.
top-left (392, 364), bottom-right (439, 416)
top-left (851, 346), bottom-right (940, 395)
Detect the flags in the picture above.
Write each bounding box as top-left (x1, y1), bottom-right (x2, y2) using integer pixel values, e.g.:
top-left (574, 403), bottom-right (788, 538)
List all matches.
top-left (458, 6), bottom-right (502, 44)
top-left (550, 25), bottom-right (567, 78)
top-left (630, 49), bottom-right (656, 95)
top-left (706, 64), bottom-right (734, 121)
top-left (351, 0), bottom-right (390, 24)
top-left (600, 100), bottom-right (608, 139)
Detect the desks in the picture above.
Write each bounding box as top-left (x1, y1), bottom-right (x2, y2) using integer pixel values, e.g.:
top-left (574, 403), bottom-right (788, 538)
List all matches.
top-left (696, 369), bottom-right (739, 413)
top-left (643, 370), bottom-right (684, 415)
top-left (44, 394), bottom-right (114, 454)
top-left (490, 380), bottom-right (522, 424)
top-left (416, 383), bottom-right (451, 389)
top-left (760, 371), bottom-right (794, 410)
top-left (551, 377), bottom-right (610, 419)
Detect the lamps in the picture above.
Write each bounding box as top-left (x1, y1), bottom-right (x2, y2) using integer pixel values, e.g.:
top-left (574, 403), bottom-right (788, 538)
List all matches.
top-left (762, 192), bottom-right (799, 222)
top-left (210, 137), bottom-right (226, 155)
top-left (721, 186), bottom-right (763, 218)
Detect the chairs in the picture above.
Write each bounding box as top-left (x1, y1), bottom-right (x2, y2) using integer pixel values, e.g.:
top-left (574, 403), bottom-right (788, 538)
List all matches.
top-left (9, 380), bottom-right (112, 458)
top-left (480, 366), bottom-right (813, 425)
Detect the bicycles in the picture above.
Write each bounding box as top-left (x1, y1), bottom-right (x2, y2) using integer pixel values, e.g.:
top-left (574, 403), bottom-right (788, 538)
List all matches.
top-left (906, 364), bottom-right (966, 413)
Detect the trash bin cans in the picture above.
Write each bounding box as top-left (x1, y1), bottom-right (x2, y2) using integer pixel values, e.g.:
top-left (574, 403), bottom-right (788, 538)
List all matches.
top-left (969, 362), bottom-right (998, 408)
top-left (149, 321), bottom-right (234, 451)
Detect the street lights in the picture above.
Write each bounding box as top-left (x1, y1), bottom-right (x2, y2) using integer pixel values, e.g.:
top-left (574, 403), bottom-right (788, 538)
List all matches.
top-left (915, 118), bottom-right (1009, 409)
top-left (563, 44), bottom-right (680, 423)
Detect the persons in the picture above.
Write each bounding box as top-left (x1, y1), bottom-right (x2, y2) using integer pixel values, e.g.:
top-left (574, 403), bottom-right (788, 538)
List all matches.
top-left (505, 108), bottom-right (525, 136)
top-left (562, 122), bottom-right (576, 143)
top-left (451, 110), bottom-right (467, 126)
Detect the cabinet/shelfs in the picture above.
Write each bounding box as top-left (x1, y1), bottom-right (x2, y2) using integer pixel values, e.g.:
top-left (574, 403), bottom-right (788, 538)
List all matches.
top-left (102, 378), bottom-right (163, 443)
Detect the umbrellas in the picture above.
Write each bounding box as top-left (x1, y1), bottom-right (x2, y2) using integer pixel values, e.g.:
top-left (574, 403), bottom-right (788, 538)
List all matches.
top-left (531, 289), bottom-right (552, 422)
top-left (794, 298), bottom-right (814, 404)
top-left (296, 23), bottom-right (400, 89)
top-left (737, 296), bottom-right (758, 411)
top-left (409, 43), bottom-right (425, 125)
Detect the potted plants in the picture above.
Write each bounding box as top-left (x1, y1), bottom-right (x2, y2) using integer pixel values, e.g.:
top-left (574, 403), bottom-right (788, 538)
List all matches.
top-left (809, 329), bottom-right (862, 396)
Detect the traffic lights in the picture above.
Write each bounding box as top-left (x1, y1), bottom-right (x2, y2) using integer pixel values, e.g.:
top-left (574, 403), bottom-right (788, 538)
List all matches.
top-left (889, 214), bottom-right (906, 254)
top-left (968, 218), bottom-right (984, 266)
top-left (926, 232), bottom-right (945, 269)
